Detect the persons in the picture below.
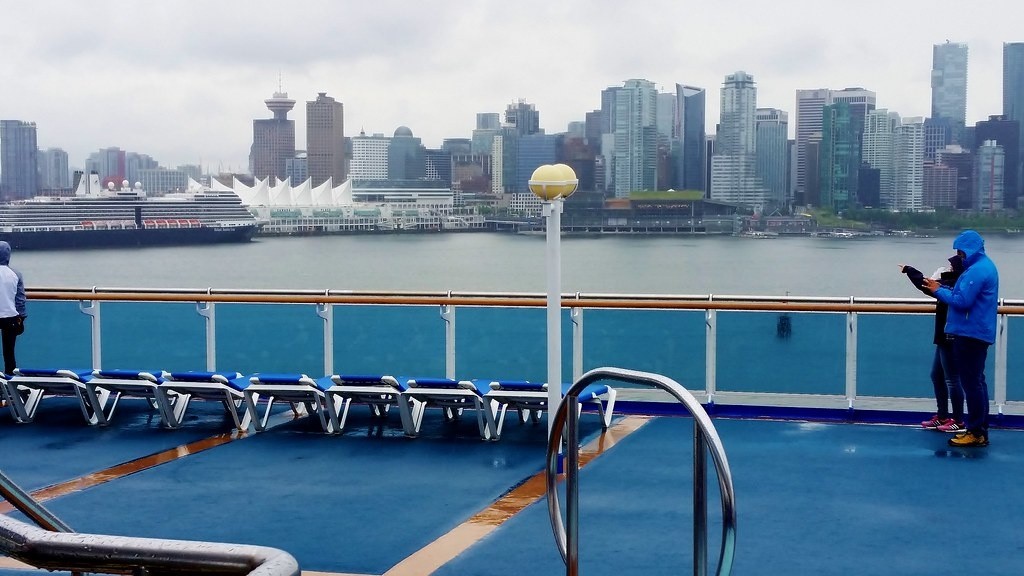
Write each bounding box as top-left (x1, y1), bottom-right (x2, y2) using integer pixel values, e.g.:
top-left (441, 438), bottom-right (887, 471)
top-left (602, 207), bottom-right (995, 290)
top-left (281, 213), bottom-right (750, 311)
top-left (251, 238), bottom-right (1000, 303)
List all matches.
top-left (897, 255), bottom-right (967, 433)
top-left (0, 241), bottom-right (29, 376)
top-left (921, 230), bottom-right (998, 447)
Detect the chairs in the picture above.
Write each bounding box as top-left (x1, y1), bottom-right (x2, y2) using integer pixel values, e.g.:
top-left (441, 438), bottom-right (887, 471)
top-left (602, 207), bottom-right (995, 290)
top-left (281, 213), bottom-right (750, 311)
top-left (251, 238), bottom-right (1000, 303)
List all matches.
top-left (0, 365), bottom-right (617, 444)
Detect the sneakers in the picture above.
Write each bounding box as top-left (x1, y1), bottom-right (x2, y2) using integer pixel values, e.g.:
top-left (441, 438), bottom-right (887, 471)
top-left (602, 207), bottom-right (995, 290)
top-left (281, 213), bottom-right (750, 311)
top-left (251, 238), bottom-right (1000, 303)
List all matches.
top-left (921, 415), bottom-right (951, 429)
top-left (937, 419), bottom-right (968, 433)
top-left (948, 431), bottom-right (991, 447)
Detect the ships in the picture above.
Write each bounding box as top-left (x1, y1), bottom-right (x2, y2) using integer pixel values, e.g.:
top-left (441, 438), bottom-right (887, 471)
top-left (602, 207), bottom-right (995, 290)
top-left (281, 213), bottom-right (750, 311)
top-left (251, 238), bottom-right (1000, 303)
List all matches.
top-left (0, 188), bottom-right (260, 251)
